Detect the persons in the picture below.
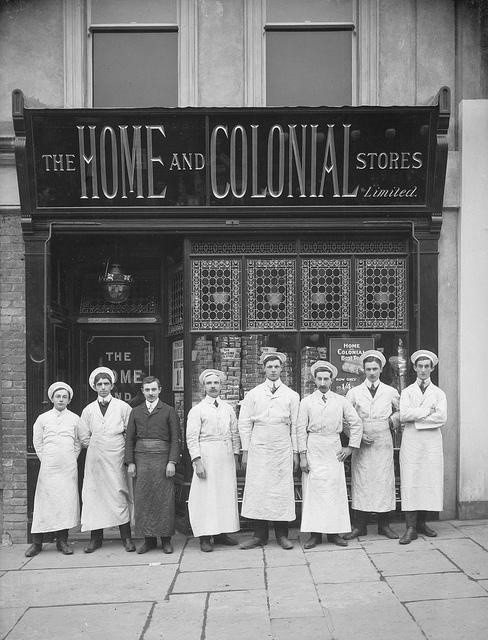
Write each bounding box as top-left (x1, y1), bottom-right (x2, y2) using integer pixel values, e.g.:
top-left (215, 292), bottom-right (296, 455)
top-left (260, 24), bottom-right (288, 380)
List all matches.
top-left (239, 351), bottom-right (300, 548)
top-left (124, 377), bottom-right (180, 554)
top-left (398, 349), bottom-right (448, 545)
top-left (79, 366), bottom-right (136, 552)
top-left (297, 360), bottom-right (364, 549)
top-left (341, 349), bottom-right (404, 541)
top-left (185, 369), bottom-right (242, 552)
top-left (25, 382), bottom-right (80, 556)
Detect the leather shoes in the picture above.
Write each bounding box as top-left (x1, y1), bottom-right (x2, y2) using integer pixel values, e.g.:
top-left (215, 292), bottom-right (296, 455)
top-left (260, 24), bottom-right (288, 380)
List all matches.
top-left (278, 536), bottom-right (293, 549)
top-left (239, 537), bottom-right (267, 549)
top-left (327, 534), bottom-right (347, 545)
top-left (418, 524), bottom-right (437, 537)
top-left (344, 528), bottom-right (367, 540)
top-left (56, 540), bottom-right (73, 555)
top-left (399, 527), bottom-right (417, 544)
top-left (25, 543), bottom-right (42, 557)
top-left (200, 534), bottom-right (237, 552)
top-left (84, 539), bottom-right (102, 553)
top-left (378, 526), bottom-right (399, 538)
top-left (304, 534), bottom-right (322, 549)
top-left (123, 537), bottom-right (172, 554)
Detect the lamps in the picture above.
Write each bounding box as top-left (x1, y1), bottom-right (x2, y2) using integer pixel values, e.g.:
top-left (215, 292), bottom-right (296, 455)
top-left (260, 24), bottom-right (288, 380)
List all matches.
top-left (98, 258), bottom-right (134, 304)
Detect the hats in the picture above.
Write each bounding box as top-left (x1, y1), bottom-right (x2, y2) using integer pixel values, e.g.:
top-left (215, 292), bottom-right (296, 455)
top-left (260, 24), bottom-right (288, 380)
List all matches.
top-left (199, 369), bottom-right (225, 386)
top-left (258, 352), bottom-right (286, 364)
top-left (47, 382), bottom-right (74, 402)
top-left (411, 349), bottom-right (439, 366)
top-left (311, 360), bottom-right (338, 380)
top-left (88, 366), bottom-right (114, 391)
top-left (360, 350), bottom-right (386, 368)
